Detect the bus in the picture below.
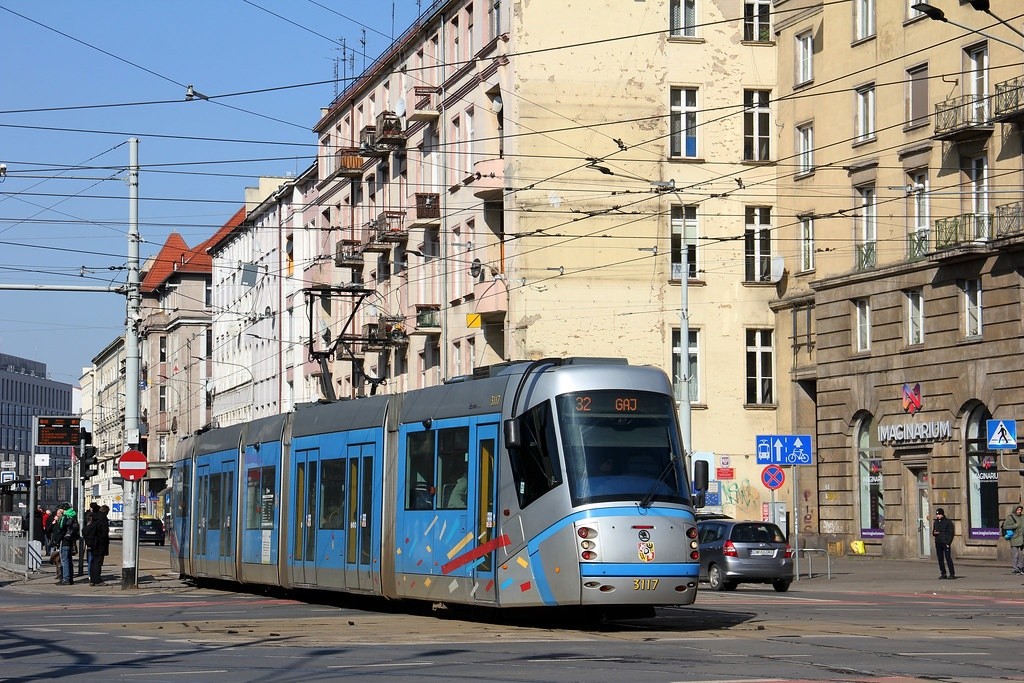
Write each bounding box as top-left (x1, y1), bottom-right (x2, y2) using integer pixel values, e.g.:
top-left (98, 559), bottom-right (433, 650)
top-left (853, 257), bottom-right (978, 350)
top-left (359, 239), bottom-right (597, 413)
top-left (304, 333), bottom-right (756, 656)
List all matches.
top-left (167, 283), bottom-right (711, 624)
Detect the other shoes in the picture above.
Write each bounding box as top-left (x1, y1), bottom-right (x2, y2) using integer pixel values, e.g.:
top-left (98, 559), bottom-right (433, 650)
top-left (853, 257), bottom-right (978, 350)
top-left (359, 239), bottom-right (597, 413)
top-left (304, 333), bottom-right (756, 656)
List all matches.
top-left (948, 574), bottom-right (954, 580)
top-left (1019, 568), bottom-right (1024, 574)
top-left (89, 582), bottom-right (108, 586)
top-left (56, 580), bottom-right (74, 586)
top-left (54, 577), bottom-right (61, 580)
top-left (938, 574), bottom-right (947, 579)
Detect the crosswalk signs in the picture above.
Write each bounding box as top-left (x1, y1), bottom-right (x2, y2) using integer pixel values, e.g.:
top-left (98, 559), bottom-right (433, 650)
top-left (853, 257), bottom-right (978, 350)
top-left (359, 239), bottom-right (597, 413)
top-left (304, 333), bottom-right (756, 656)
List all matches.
top-left (985, 418), bottom-right (1018, 449)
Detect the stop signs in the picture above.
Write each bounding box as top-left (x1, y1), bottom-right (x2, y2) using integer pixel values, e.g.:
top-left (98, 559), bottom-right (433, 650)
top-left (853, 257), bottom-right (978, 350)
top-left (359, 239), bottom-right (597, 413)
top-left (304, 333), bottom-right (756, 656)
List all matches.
top-left (117, 450), bottom-right (149, 481)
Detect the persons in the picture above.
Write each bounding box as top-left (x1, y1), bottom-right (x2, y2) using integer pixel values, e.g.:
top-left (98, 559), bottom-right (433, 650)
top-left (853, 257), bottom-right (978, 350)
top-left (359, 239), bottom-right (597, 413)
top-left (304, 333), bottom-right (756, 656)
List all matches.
top-left (1003, 504), bottom-right (1024, 575)
top-left (25, 505), bottom-right (57, 556)
top-left (46, 502), bottom-right (80, 586)
top-left (82, 502), bottom-right (110, 586)
top-left (447, 471), bottom-right (468, 507)
top-left (933, 508), bottom-right (955, 579)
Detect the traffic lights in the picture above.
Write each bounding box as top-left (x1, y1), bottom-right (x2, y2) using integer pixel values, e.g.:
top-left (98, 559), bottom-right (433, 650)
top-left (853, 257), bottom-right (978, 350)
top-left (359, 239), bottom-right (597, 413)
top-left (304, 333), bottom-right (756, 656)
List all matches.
top-left (86, 446), bottom-right (98, 480)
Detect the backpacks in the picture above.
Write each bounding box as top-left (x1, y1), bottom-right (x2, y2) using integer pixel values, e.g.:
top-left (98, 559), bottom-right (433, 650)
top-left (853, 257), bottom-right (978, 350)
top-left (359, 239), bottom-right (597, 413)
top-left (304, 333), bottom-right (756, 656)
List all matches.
top-left (1001, 516), bottom-right (1017, 540)
top-left (83, 518), bottom-right (103, 546)
top-left (64, 515), bottom-right (78, 540)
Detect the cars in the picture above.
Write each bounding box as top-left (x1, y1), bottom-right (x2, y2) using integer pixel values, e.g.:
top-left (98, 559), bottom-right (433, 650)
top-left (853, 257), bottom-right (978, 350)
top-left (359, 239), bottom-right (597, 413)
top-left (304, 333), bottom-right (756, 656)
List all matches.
top-left (696, 519), bottom-right (794, 592)
top-left (136, 517), bottom-right (165, 546)
top-left (107, 519), bottom-right (123, 540)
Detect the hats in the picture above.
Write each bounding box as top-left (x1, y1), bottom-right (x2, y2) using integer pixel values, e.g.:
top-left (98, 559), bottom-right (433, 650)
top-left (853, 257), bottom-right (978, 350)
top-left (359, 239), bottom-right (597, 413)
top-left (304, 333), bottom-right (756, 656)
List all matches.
top-left (936, 508), bottom-right (944, 515)
